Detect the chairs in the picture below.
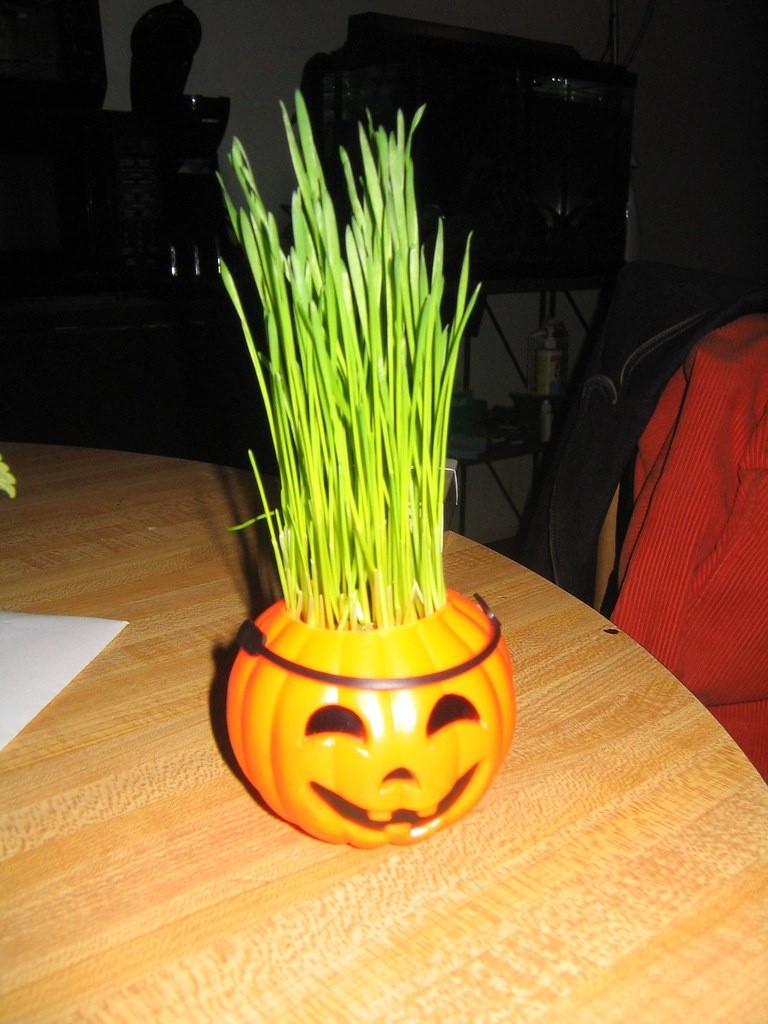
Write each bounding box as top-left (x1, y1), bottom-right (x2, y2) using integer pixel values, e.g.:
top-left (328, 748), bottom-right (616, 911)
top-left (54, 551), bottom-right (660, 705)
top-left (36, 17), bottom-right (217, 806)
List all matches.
top-left (519, 258), bottom-right (768, 784)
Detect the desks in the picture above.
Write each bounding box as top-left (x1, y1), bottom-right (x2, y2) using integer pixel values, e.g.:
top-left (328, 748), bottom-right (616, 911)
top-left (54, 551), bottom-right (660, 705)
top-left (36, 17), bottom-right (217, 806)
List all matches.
top-left (0, 443), bottom-right (768, 1024)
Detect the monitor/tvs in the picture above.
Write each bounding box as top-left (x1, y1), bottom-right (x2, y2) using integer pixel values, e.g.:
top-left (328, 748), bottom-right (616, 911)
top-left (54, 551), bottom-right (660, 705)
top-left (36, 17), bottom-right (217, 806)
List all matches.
top-left (338, 13), bottom-right (639, 292)
